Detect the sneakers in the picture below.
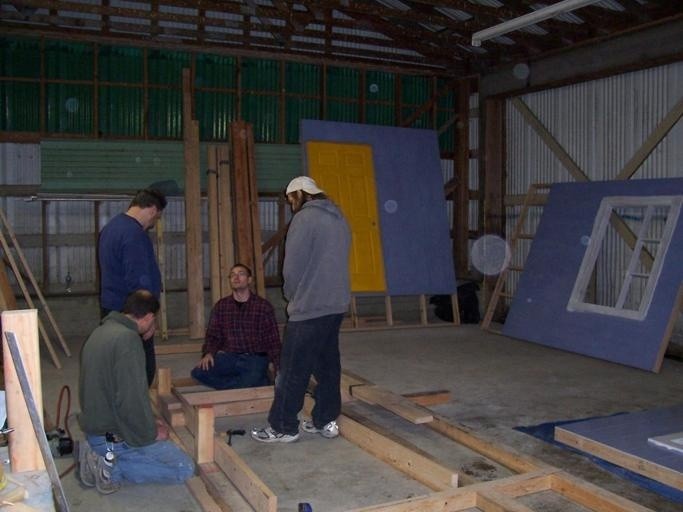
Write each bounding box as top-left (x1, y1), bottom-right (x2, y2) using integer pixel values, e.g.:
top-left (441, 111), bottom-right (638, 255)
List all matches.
top-left (87, 449), bottom-right (116, 494)
top-left (251, 426), bottom-right (299, 443)
top-left (302, 420), bottom-right (339, 438)
top-left (79, 441), bottom-right (95, 488)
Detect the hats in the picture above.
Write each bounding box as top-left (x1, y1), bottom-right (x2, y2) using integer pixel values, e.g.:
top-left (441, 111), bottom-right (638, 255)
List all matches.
top-left (286, 176), bottom-right (324, 195)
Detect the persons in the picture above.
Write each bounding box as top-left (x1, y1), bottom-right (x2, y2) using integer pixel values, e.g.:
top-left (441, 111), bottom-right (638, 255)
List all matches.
top-left (97, 189), bottom-right (166, 390)
top-left (250, 176), bottom-right (350, 442)
top-left (191, 262), bottom-right (282, 387)
top-left (74, 290), bottom-right (195, 493)
